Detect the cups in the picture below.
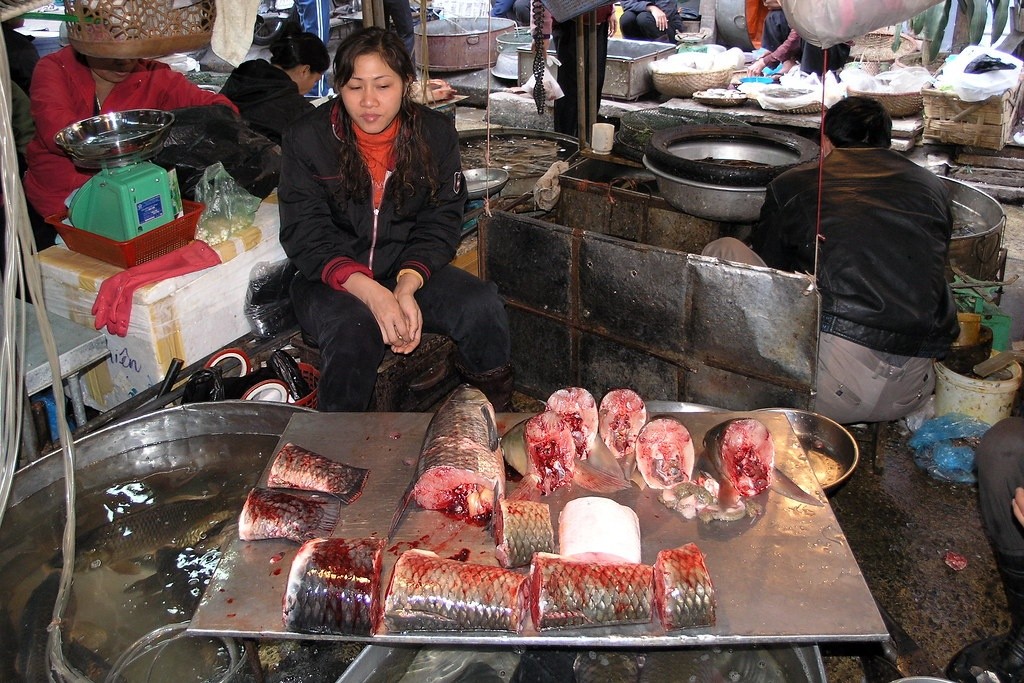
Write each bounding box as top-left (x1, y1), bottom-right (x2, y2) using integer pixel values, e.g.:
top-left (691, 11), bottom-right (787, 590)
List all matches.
top-left (591, 123), bottom-right (614, 155)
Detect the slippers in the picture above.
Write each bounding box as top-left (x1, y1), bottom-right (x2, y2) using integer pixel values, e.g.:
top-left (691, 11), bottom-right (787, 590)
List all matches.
top-left (768, 73), bottom-right (785, 85)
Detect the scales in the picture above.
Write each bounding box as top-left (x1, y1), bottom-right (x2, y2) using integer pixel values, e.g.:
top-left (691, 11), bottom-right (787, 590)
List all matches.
top-left (53, 108), bottom-right (175, 243)
top-left (948, 257), bottom-right (1021, 353)
top-left (459, 166), bottom-right (510, 231)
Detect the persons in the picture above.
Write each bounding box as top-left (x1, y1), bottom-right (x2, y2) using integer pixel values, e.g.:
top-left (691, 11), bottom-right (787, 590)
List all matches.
top-left (746, 0), bottom-right (855, 84)
top-left (0, 0), bottom-right (54, 98)
top-left (383, 0), bottom-right (415, 72)
top-left (277, 26), bottom-right (516, 413)
top-left (620, 0), bottom-right (684, 45)
top-left (946, 416), bottom-right (1024, 683)
top-left (487, 0), bottom-right (531, 31)
top-left (216, 28), bottom-right (331, 152)
top-left (293, 0), bottom-right (336, 98)
top-left (530, 0), bottom-right (619, 143)
top-left (15, 45), bottom-right (238, 305)
top-left (702, 95), bottom-right (949, 428)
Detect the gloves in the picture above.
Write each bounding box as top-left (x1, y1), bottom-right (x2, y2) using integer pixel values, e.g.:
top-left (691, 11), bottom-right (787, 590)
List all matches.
top-left (91, 239), bottom-right (222, 337)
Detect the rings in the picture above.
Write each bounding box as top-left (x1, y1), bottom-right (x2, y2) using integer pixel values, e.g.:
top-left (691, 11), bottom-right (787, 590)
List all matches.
top-left (398, 335), bottom-right (403, 339)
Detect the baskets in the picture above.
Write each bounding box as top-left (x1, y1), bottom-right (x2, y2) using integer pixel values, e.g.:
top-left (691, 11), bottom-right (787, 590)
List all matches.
top-left (748, 98), bottom-right (821, 113)
top-left (921, 61), bottom-right (1024, 151)
top-left (693, 92), bottom-right (748, 107)
top-left (850, 32), bottom-right (917, 62)
top-left (45, 200), bottom-right (203, 270)
top-left (846, 86), bottom-right (924, 116)
top-left (652, 68), bottom-right (733, 98)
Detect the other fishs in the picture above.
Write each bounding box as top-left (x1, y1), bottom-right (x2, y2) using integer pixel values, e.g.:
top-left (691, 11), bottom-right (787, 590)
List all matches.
top-left (238, 381), bottom-right (826, 633)
top-left (409, 79), bottom-right (457, 104)
top-left (20, 463), bottom-right (369, 683)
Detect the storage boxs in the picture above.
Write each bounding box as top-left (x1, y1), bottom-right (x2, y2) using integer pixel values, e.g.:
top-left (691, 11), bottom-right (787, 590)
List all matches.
top-left (517, 40), bottom-right (677, 99)
top-left (32, 204), bottom-right (289, 414)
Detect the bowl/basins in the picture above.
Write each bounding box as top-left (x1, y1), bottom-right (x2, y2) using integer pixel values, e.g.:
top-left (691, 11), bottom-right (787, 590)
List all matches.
top-left (749, 407), bottom-right (859, 488)
top-left (643, 155), bottom-right (766, 220)
top-left (952, 313), bottom-right (982, 346)
top-left (739, 77), bottom-right (772, 84)
top-left (491, 46), bottom-right (519, 79)
top-left (413, 18), bottom-right (515, 72)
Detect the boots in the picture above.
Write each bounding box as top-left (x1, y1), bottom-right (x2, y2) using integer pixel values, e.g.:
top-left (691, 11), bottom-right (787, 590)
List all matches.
top-left (945, 552), bottom-right (1024, 683)
top-left (457, 358), bottom-right (524, 413)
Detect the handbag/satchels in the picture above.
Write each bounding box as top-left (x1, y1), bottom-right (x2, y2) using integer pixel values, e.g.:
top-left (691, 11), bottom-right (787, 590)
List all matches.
top-left (521, 54), bottom-right (566, 100)
top-left (193, 163), bottom-right (261, 244)
top-left (907, 413), bottom-right (992, 483)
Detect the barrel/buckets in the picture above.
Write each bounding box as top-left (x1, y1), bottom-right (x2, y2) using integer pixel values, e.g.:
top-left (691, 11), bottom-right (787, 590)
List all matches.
top-left (933, 174), bottom-right (1004, 283)
top-left (934, 347), bottom-right (1023, 428)
top-left (457, 128), bottom-right (591, 203)
top-left (0, 400), bottom-right (828, 683)
